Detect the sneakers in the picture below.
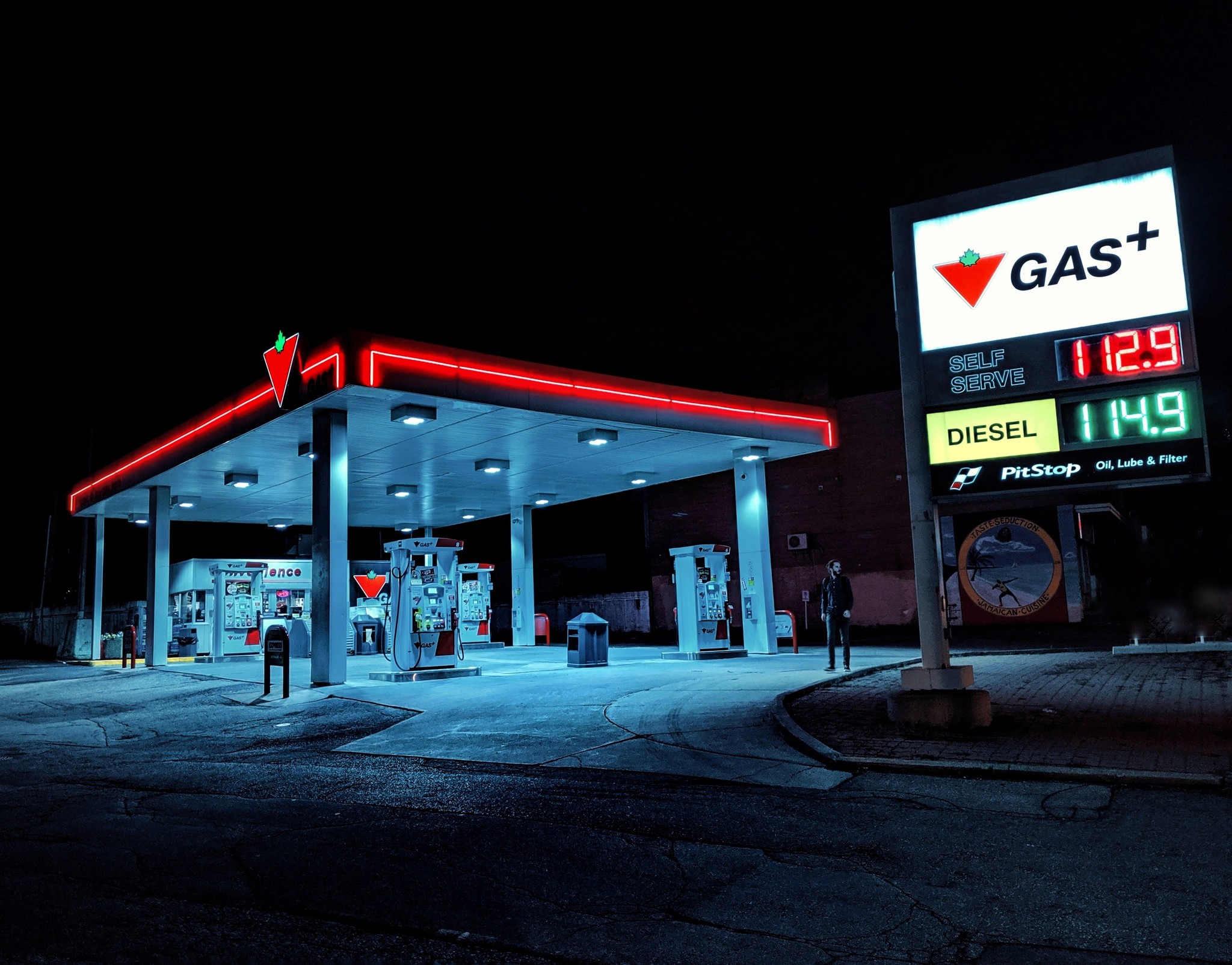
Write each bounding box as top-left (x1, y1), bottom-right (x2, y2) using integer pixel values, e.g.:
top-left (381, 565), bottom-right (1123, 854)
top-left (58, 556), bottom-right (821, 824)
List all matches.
top-left (843, 665), bottom-right (851, 672)
top-left (824, 665), bottom-right (836, 671)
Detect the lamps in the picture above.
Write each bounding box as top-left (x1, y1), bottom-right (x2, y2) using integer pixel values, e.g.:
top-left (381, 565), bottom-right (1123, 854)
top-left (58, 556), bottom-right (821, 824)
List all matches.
top-left (457, 509), bottom-right (485, 520)
top-left (390, 403), bottom-right (436, 423)
top-left (394, 523), bottom-right (420, 532)
top-left (527, 492), bottom-right (556, 505)
top-left (224, 474), bottom-right (258, 489)
top-left (387, 485), bottom-right (417, 498)
top-left (732, 447), bottom-right (768, 461)
top-left (475, 459), bottom-right (512, 473)
top-left (268, 518), bottom-right (294, 528)
top-left (578, 429), bottom-right (619, 445)
top-left (128, 513), bottom-right (148, 524)
top-left (621, 471), bottom-right (659, 484)
top-left (171, 495), bottom-right (201, 507)
top-left (297, 443), bottom-right (313, 458)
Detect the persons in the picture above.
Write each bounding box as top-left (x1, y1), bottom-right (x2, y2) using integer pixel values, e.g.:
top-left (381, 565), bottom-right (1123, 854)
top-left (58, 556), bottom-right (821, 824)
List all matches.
top-left (992, 577), bottom-right (1022, 607)
top-left (820, 559), bottom-right (854, 671)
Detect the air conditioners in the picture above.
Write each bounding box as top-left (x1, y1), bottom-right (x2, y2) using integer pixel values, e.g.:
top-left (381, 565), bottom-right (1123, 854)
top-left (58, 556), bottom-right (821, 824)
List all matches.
top-left (787, 533), bottom-right (808, 549)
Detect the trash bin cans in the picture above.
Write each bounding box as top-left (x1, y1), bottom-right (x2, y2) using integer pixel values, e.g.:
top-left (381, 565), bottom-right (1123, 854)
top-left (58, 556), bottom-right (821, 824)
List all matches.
top-left (178, 628), bottom-right (199, 657)
top-left (351, 614), bottom-right (379, 655)
top-left (566, 613), bottom-right (609, 668)
